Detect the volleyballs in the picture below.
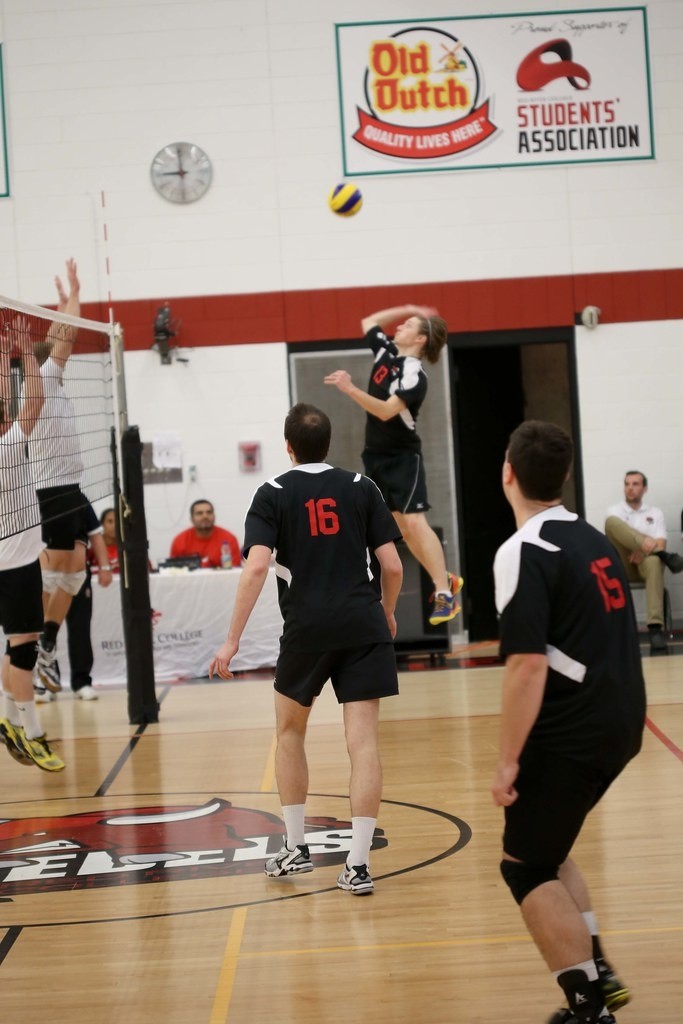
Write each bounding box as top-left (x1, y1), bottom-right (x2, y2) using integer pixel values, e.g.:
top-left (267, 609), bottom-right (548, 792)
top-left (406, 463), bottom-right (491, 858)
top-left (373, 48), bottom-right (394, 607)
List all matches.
top-left (327, 182), bottom-right (363, 217)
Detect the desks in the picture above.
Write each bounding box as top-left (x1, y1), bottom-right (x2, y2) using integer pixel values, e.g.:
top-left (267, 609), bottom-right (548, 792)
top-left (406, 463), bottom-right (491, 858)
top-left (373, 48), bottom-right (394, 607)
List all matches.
top-left (59, 568), bottom-right (282, 684)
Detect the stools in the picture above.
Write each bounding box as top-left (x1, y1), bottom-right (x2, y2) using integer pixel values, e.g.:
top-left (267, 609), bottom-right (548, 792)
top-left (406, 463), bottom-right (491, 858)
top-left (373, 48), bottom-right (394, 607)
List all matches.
top-left (629, 582), bottom-right (671, 647)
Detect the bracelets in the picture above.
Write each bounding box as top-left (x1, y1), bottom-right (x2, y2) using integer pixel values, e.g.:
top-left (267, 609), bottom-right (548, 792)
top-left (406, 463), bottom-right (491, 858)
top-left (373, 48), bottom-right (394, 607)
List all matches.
top-left (99, 565), bottom-right (111, 572)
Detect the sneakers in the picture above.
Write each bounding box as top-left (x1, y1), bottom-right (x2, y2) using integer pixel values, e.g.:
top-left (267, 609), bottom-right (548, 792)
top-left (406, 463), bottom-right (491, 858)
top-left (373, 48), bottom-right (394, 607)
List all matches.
top-left (263, 840), bottom-right (314, 878)
top-left (429, 591), bottom-right (461, 626)
top-left (335, 865), bottom-right (374, 896)
top-left (546, 969), bottom-right (617, 1024)
top-left (29, 647), bottom-right (62, 693)
top-left (589, 935), bottom-right (630, 1012)
top-left (429, 573), bottom-right (464, 603)
top-left (18, 726), bottom-right (66, 772)
top-left (0, 719), bottom-right (34, 766)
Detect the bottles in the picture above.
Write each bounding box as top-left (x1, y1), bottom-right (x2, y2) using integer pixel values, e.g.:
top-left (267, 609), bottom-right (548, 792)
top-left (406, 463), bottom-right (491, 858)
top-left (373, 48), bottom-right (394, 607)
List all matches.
top-left (221, 542), bottom-right (232, 569)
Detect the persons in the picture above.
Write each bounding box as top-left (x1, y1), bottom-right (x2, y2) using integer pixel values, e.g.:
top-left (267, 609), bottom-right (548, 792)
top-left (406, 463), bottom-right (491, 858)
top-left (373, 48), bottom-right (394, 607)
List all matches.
top-left (208, 403), bottom-right (403, 896)
top-left (88, 508), bottom-right (155, 574)
top-left (603, 469), bottom-right (683, 656)
top-left (171, 500), bottom-right (241, 570)
top-left (34, 492), bottom-right (113, 702)
top-left (490, 420), bottom-right (647, 1024)
top-left (15, 258), bottom-right (79, 695)
top-left (0, 316), bottom-right (67, 772)
top-left (322, 301), bottom-right (465, 625)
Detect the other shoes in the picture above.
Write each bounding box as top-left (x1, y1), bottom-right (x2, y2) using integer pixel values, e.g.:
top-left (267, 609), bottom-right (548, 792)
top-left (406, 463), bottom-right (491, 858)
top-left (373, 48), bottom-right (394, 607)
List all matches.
top-left (666, 553), bottom-right (683, 574)
top-left (35, 690), bottom-right (58, 703)
top-left (649, 632), bottom-right (668, 656)
top-left (76, 686), bottom-right (98, 700)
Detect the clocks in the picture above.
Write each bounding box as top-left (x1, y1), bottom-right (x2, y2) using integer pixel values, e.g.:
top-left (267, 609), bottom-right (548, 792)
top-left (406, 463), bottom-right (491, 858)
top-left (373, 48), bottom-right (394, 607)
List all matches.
top-left (150, 144), bottom-right (213, 205)
